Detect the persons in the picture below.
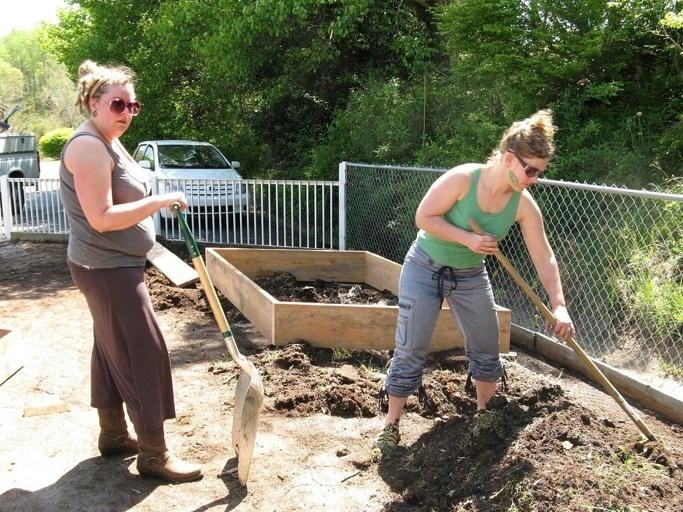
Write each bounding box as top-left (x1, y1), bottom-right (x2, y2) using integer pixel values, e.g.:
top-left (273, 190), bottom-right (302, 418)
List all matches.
top-left (59, 59), bottom-right (204, 483)
top-left (377, 109), bottom-right (577, 458)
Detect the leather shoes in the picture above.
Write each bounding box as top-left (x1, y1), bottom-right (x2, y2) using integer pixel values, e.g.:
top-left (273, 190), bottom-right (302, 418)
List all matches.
top-left (97, 434), bottom-right (138, 456)
top-left (135, 450), bottom-right (204, 484)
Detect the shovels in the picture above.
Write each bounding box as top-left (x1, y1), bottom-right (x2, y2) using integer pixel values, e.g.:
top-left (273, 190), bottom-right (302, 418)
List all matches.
top-left (171, 201), bottom-right (265, 486)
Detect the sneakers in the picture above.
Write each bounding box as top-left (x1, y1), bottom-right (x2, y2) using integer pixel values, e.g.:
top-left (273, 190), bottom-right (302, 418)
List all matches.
top-left (370, 422), bottom-right (400, 464)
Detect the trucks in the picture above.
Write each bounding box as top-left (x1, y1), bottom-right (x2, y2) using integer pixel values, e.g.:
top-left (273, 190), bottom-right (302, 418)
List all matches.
top-left (0, 133), bottom-right (40, 215)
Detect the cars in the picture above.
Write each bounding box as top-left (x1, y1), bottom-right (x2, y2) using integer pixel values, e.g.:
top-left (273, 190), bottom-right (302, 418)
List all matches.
top-left (130, 139), bottom-right (251, 229)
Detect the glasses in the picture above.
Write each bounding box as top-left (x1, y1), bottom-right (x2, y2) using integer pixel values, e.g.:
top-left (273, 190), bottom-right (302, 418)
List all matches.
top-left (95, 99), bottom-right (141, 117)
top-left (505, 150), bottom-right (544, 179)
